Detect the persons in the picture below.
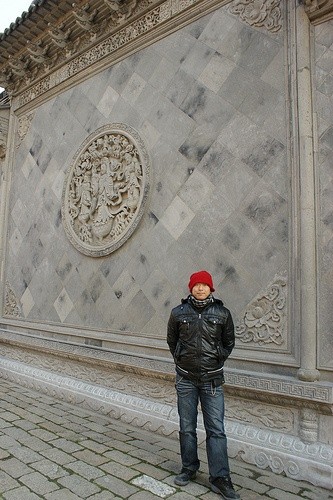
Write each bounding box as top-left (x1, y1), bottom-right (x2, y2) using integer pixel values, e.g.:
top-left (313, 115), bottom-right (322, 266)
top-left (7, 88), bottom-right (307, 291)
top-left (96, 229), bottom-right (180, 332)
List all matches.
top-left (166, 271), bottom-right (241, 500)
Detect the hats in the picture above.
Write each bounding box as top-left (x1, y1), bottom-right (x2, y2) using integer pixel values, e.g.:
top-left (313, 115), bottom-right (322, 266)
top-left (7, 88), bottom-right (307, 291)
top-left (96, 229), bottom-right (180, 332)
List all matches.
top-left (189, 271), bottom-right (217, 294)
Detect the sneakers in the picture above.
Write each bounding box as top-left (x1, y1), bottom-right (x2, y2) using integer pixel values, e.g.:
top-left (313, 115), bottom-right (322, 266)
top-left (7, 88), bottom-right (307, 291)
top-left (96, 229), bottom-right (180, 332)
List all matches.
top-left (174, 465), bottom-right (197, 486)
top-left (208, 472), bottom-right (241, 500)
